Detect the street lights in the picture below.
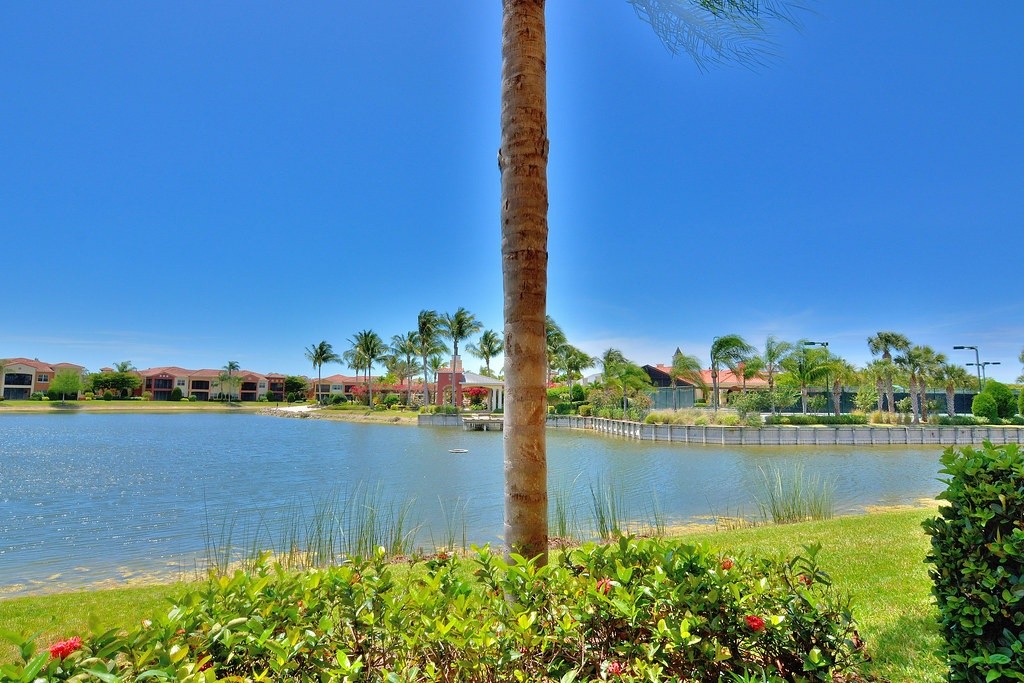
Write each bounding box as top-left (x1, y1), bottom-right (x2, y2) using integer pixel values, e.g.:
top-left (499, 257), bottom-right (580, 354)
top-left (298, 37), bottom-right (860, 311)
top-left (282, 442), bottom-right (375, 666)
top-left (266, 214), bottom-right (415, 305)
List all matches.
top-left (953, 345), bottom-right (981, 393)
top-left (804, 341), bottom-right (830, 416)
top-left (965, 361), bottom-right (1000, 383)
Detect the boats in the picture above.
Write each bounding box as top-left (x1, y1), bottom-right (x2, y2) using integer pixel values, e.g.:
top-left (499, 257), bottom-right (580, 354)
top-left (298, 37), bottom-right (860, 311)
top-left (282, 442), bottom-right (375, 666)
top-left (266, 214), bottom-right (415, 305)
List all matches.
top-left (449, 449), bottom-right (468, 453)
